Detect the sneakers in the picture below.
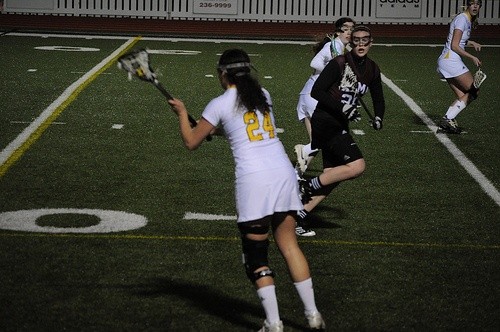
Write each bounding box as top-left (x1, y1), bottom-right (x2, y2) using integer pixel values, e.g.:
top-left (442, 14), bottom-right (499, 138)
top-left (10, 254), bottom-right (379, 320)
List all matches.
top-left (294, 162), bottom-right (306, 182)
top-left (294, 144), bottom-right (309, 175)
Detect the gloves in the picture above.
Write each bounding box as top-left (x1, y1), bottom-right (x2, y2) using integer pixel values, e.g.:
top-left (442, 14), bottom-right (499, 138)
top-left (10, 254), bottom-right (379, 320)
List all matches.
top-left (343, 102), bottom-right (361, 123)
top-left (370, 117), bottom-right (383, 130)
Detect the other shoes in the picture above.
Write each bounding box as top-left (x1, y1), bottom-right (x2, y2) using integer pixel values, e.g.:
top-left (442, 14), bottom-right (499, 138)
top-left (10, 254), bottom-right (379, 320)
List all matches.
top-left (258, 320), bottom-right (284, 332)
top-left (437, 126), bottom-right (446, 133)
top-left (306, 312), bottom-right (327, 331)
top-left (295, 216), bottom-right (317, 237)
top-left (439, 115), bottom-right (461, 134)
top-left (296, 179), bottom-right (304, 202)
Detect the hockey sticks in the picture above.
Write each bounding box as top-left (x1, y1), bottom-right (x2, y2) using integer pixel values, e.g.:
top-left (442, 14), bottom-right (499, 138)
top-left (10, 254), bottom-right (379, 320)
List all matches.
top-left (473, 47), bottom-right (487, 89)
top-left (326, 34), bottom-right (339, 58)
top-left (341, 63), bottom-right (376, 122)
top-left (117, 48), bottom-right (212, 142)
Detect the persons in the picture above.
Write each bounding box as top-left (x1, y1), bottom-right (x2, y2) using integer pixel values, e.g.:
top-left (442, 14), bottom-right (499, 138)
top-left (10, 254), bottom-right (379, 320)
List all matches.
top-left (293, 17), bottom-right (356, 178)
top-left (291, 26), bottom-right (385, 238)
top-left (168, 49), bottom-right (328, 332)
top-left (435, 1), bottom-right (482, 135)
